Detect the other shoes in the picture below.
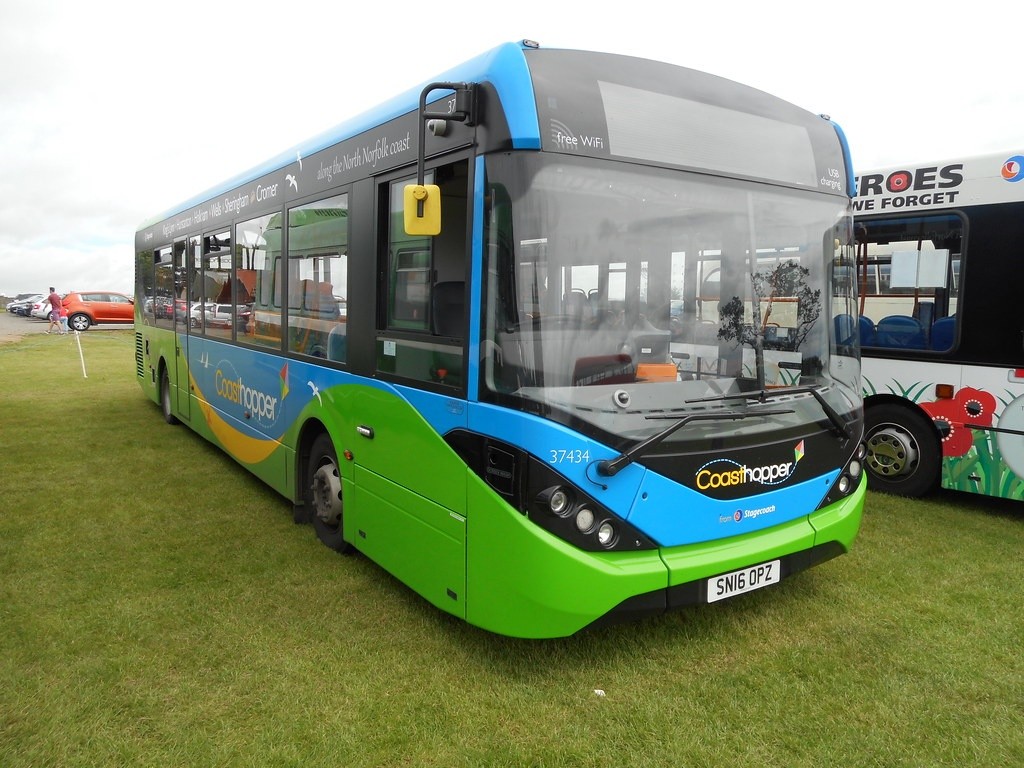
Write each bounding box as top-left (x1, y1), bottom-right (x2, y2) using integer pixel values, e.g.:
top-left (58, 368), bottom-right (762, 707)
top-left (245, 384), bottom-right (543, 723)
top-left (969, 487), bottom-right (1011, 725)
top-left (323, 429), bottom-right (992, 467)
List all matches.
top-left (56, 331), bottom-right (64, 335)
top-left (45, 330), bottom-right (51, 334)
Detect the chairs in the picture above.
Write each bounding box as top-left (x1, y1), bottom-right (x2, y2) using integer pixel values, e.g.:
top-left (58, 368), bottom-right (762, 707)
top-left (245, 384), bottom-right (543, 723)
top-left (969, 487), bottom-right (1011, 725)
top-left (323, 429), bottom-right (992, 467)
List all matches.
top-left (931, 317), bottom-right (958, 351)
top-left (564, 289), bottom-right (586, 308)
top-left (296, 279), bottom-right (318, 314)
top-left (761, 323), bottom-right (779, 338)
top-left (877, 315), bottom-right (928, 349)
top-left (691, 320), bottom-right (717, 345)
top-left (326, 325), bottom-right (348, 362)
top-left (588, 288), bottom-right (606, 304)
top-left (307, 282), bottom-right (340, 317)
top-left (833, 314), bottom-right (877, 346)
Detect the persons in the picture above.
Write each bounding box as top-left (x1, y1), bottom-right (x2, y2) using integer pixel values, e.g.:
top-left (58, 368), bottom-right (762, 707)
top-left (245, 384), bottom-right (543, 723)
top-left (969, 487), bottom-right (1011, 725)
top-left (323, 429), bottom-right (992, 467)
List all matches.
top-left (43, 287), bottom-right (64, 335)
top-left (58, 306), bottom-right (69, 335)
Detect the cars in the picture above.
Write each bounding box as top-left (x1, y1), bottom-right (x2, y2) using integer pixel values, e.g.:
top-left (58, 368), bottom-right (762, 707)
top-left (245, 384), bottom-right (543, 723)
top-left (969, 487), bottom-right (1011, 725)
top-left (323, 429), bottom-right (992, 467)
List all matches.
top-left (142, 294), bottom-right (256, 334)
top-left (5, 290), bottom-right (135, 331)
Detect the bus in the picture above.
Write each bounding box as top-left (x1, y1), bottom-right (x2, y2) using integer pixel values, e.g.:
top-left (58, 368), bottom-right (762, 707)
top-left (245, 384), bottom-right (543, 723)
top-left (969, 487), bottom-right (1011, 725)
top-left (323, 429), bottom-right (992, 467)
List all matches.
top-left (634, 145), bottom-right (1024, 501)
top-left (135, 39), bottom-right (869, 638)
